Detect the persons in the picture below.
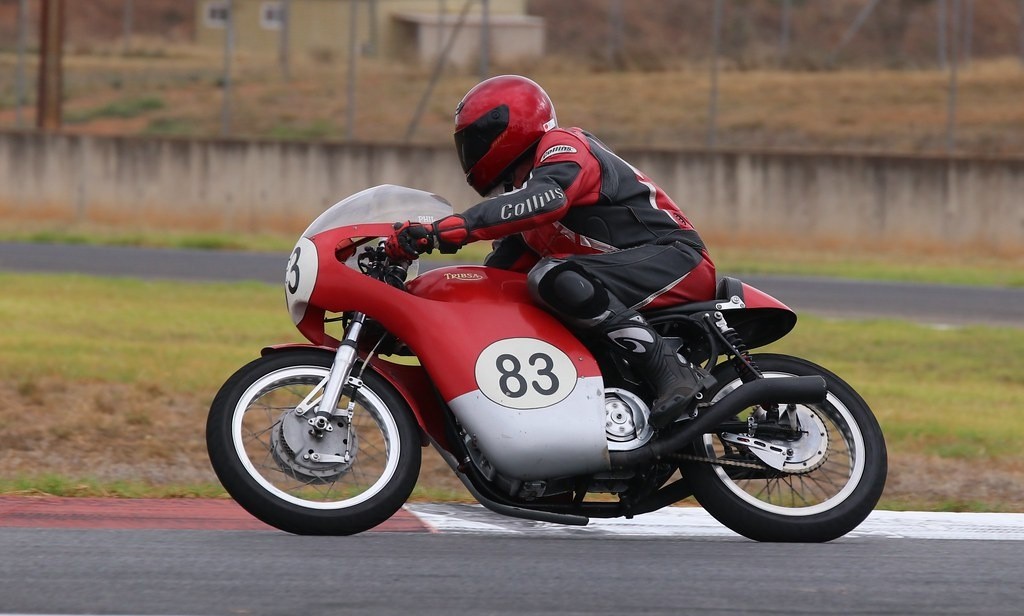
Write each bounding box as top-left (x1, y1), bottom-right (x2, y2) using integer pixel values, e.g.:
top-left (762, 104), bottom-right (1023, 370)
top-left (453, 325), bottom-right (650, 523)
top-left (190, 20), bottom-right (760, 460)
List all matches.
top-left (383, 76), bottom-right (717, 428)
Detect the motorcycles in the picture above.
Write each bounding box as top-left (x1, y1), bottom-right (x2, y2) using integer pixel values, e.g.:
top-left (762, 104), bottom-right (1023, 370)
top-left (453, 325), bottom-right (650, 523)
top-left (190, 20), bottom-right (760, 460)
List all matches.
top-left (203, 183), bottom-right (888, 548)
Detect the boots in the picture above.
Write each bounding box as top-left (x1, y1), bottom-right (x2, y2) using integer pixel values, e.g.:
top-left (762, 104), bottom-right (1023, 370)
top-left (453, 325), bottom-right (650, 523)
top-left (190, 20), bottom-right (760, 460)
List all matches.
top-left (603, 312), bottom-right (720, 428)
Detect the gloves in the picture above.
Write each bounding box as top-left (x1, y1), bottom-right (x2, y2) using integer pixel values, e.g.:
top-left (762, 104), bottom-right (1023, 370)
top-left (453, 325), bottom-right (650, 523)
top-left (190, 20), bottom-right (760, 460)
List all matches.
top-left (387, 214), bottom-right (466, 261)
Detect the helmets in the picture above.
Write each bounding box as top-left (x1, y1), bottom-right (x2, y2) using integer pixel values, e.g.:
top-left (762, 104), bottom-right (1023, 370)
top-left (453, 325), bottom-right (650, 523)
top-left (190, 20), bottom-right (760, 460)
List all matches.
top-left (454, 73), bottom-right (559, 197)
